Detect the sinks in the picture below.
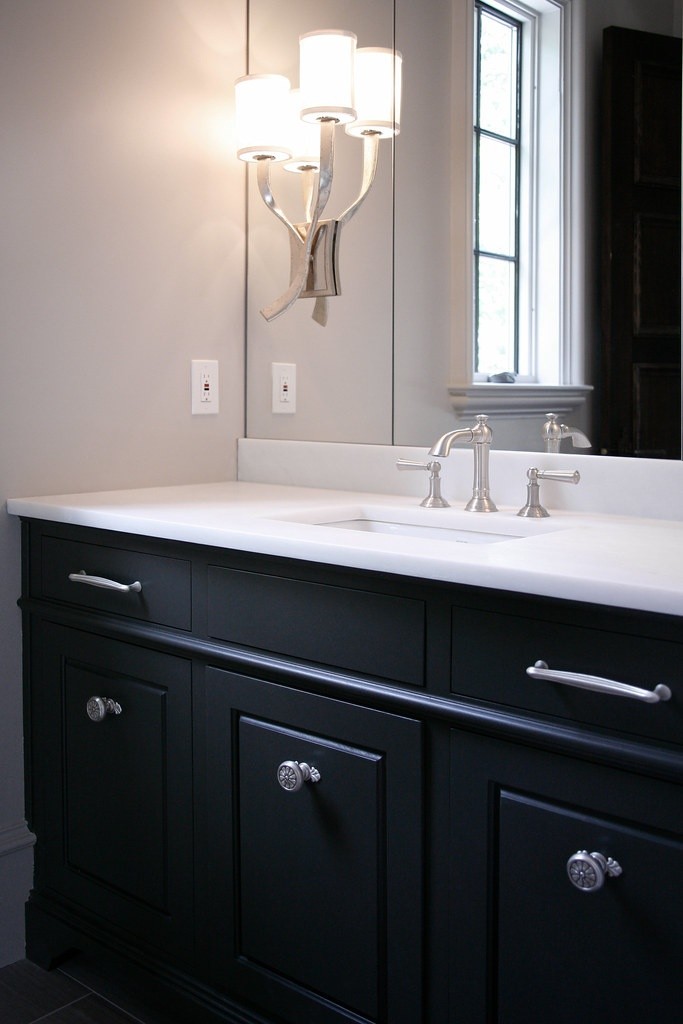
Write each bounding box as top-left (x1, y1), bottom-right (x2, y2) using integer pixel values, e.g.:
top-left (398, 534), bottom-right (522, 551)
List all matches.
top-left (263, 502), bottom-right (571, 548)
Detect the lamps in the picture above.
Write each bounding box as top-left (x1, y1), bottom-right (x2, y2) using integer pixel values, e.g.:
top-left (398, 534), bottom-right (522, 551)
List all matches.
top-left (234, 30), bottom-right (357, 319)
top-left (280, 45), bottom-right (404, 327)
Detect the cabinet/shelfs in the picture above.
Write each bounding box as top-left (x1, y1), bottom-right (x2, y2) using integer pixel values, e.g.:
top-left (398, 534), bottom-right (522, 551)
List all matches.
top-left (428, 568), bottom-right (683, 1024)
top-left (13, 512), bottom-right (195, 1023)
top-left (197, 527), bottom-right (429, 1024)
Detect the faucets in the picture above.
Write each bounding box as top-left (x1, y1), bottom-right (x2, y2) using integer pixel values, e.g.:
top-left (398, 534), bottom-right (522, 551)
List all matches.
top-left (426, 414), bottom-right (499, 513)
top-left (540, 412), bottom-right (592, 454)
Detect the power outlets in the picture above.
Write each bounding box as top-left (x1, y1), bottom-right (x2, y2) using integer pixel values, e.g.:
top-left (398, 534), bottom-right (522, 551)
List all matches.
top-left (191, 360), bottom-right (219, 415)
top-left (272, 362), bottom-right (295, 413)
